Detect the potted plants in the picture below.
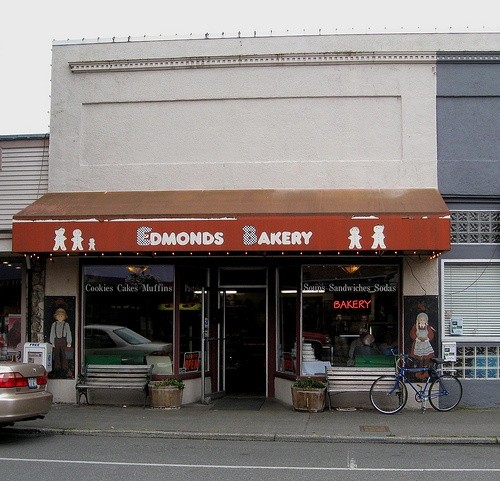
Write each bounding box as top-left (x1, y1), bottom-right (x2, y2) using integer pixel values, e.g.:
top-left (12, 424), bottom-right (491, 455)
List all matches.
top-left (291, 379), bottom-right (329, 413)
top-left (148, 378), bottom-right (186, 410)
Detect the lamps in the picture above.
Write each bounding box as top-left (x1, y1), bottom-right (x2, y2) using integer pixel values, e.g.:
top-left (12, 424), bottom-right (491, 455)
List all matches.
top-left (341, 266), bottom-right (360, 273)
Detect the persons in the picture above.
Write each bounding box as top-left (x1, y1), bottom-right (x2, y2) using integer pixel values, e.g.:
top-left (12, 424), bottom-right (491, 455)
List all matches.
top-left (348, 327), bottom-right (398, 357)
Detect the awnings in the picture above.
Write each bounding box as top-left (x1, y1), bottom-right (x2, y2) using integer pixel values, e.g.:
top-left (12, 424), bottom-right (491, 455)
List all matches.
top-left (12, 187), bottom-right (451, 258)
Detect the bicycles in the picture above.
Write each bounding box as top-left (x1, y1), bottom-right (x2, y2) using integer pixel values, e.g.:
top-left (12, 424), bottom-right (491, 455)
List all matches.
top-left (368, 349), bottom-right (463, 415)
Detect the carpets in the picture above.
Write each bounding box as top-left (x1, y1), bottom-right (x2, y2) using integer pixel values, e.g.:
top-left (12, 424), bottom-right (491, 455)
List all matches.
top-left (208, 398), bottom-right (265, 410)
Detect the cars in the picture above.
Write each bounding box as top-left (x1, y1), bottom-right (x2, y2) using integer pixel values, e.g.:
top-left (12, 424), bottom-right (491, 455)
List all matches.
top-left (83, 325), bottom-right (173, 365)
top-left (302, 331), bottom-right (331, 358)
top-left (0, 361), bottom-right (54, 425)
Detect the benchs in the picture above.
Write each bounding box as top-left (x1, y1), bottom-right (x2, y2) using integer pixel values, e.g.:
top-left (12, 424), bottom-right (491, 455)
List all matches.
top-left (75, 364), bottom-right (154, 409)
